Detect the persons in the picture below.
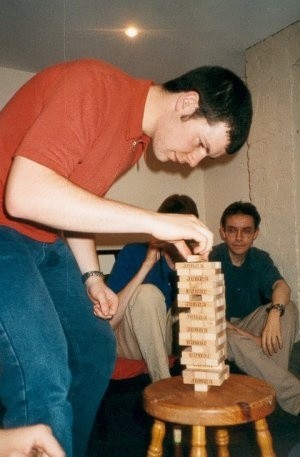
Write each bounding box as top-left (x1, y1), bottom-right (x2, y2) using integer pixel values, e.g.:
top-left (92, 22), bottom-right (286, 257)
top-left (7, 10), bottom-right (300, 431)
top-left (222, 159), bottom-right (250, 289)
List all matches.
top-left (207, 201), bottom-right (300, 416)
top-left (101, 194), bottom-right (204, 383)
top-left (0, 57), bottom-right (253, 457)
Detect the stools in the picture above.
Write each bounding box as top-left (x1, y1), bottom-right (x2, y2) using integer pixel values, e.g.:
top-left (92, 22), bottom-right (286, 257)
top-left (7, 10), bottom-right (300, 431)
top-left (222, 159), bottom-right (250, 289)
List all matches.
top-left (142, 372), bottom-right (278, 456)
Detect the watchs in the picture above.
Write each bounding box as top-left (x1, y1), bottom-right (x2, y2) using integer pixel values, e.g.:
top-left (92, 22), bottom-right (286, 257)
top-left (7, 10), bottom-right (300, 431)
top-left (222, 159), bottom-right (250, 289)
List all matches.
top-left (267, 303), bottom-right (285, 316)
top-left (82, 271), bottom-right (105, 283)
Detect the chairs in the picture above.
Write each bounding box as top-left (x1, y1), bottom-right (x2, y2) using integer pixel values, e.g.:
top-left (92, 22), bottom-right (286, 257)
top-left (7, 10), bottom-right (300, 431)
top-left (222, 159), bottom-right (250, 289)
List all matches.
top-left (98, 249), bottom-right (179, 424)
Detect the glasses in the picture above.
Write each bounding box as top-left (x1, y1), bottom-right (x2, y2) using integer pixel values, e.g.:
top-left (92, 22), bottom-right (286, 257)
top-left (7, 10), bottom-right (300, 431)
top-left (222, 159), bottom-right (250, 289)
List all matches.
top-left (226, 228), bottom-right (256, 238)
